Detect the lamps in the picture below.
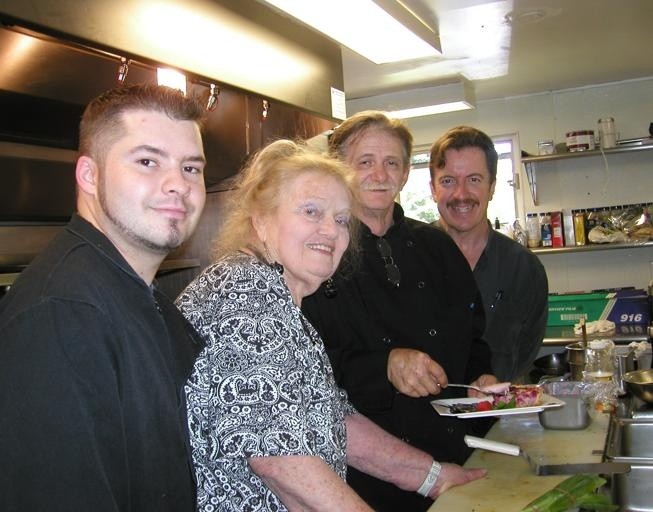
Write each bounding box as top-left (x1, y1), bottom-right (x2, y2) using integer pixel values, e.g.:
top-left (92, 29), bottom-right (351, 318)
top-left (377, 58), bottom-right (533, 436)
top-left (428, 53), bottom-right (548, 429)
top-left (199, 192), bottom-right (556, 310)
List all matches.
top-left (345, 80), bottom-right (476, 123)
top-left (254, 0), bottom-right (443, 67)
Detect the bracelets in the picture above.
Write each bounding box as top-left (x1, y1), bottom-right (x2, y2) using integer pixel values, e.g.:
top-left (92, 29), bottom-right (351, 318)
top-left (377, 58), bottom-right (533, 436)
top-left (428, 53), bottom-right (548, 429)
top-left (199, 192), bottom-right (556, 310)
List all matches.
top-left (414, 461), bottom-right (442, 500)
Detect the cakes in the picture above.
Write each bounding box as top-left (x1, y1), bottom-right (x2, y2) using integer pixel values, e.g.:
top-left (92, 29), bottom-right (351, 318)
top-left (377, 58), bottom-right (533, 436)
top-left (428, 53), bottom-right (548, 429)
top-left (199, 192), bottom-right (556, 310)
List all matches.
top-left (492, 384), bottom-right (543, 407)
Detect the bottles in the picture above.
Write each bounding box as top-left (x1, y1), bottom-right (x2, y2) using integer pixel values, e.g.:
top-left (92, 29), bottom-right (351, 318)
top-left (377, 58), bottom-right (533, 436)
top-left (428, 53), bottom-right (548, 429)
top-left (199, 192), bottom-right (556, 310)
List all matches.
top-left (526, 211), bottom-right (552, 247)
top-left (598, 117), bottom-right (616, 149)
top-left (590, 340), bottom-right (617, 411)
top-left (571, 203), bottom-right (609, 245)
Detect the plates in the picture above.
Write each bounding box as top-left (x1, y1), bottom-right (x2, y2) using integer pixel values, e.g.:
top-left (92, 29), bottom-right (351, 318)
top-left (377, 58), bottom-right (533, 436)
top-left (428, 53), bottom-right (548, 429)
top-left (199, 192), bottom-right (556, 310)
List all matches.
top-left (429, 392), bottom-right (565, 419)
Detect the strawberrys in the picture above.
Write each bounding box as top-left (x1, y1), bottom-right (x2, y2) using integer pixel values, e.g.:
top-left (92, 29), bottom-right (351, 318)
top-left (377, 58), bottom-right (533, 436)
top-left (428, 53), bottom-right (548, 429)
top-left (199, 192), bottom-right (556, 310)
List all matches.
top-left (475, 401), bottom-right (492, 411)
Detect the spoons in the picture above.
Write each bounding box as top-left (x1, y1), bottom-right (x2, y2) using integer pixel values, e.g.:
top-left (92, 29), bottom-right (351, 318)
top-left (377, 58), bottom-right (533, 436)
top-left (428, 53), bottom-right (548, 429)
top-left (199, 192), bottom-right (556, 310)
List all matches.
top-left (447, 383), bottom-right (510, 393)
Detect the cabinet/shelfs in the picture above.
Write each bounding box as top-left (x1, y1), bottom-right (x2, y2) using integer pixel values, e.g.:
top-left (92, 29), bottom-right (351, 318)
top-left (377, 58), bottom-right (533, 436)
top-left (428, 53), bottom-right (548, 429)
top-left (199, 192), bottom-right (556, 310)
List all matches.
top-left (519, 135), bottom-right (653, 256)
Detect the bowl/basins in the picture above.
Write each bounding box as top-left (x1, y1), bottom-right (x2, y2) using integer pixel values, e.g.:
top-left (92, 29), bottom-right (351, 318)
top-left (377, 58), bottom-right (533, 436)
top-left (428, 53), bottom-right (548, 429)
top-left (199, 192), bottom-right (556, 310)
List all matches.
top-left (622, 368), bottom-right (652, 403)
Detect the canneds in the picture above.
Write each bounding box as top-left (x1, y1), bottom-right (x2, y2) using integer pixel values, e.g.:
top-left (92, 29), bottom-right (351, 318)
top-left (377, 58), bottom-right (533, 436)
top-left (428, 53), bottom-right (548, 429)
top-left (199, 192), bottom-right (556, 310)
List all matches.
top-left (526, 202), bottom-right (653, 248)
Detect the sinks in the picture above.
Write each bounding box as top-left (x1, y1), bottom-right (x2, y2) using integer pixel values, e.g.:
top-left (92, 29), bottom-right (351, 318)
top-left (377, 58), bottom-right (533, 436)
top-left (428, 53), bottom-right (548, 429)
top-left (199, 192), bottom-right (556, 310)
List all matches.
top-left (604, 414), bottom-right (653, 459)
top-left (595, 459), bottom-right (653, 512)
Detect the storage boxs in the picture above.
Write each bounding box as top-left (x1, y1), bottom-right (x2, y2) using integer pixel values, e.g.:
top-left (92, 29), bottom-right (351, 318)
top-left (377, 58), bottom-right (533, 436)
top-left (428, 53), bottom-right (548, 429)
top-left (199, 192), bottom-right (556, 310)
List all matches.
top-left (546, 290), bottom-right (650, 327)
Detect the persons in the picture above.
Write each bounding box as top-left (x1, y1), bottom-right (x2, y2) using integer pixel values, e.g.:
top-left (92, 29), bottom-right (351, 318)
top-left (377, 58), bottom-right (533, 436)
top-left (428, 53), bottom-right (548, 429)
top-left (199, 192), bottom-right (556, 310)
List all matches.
top-left (1, 80), bottom-right (211, 512)
top-left (298, 110), bottom-right (499, 510)
top-left (422, 126), bottom-right (551, 464)
top-left (171, 137), bottom-right (490, 511)
